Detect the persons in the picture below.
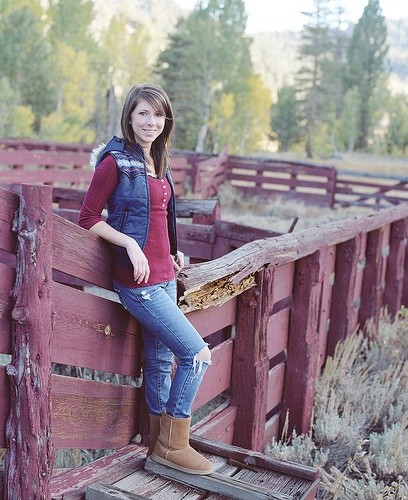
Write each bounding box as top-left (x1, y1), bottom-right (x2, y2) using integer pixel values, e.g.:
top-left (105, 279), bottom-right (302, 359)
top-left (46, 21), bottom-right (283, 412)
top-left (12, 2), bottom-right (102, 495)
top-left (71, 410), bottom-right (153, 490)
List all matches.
top-left (77, 82), bottom-right (216, 477)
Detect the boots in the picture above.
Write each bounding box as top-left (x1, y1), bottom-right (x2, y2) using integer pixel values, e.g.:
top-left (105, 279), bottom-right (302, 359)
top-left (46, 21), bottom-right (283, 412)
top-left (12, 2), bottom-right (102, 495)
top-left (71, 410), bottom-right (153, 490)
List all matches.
top-left (147, 413), bottom-right (161, 457)
top-left (152, 412), bottom-right (214, 474)
